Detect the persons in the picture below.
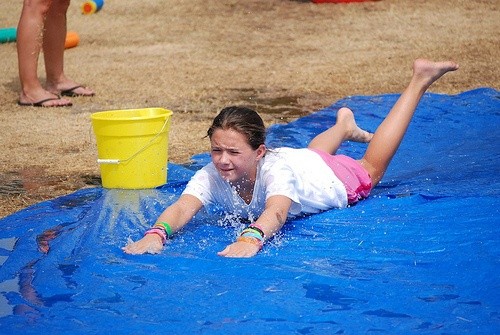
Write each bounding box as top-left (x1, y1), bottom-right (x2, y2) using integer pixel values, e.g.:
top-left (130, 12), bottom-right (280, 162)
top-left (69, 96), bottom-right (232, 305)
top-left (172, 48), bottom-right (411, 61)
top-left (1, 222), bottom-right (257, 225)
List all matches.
top-left (121, 59), bottom-right (460, 258)
top-left (15, 0), bottom-right (97, 107)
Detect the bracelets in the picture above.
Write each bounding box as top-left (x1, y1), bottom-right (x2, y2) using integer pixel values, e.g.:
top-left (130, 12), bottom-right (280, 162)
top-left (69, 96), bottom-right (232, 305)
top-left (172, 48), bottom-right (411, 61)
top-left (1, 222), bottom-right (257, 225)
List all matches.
top-left (143, 222), bottom-right (173, 245)
top-left (237, 223), bottom-right (267, 251)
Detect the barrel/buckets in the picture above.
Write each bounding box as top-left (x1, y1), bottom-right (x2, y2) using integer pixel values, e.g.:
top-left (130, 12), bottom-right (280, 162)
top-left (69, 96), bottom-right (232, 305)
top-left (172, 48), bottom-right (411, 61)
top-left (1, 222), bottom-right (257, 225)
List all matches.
top-left (89, 108), bottom-right (174, 190)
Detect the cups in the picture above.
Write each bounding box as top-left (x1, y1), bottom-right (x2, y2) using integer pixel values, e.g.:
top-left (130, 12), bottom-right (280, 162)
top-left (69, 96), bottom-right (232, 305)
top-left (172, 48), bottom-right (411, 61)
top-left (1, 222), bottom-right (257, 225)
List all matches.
top-left (0, 27), bottom-right (17, 43)
top-left (80, 0), bottom-right (104, 15)
top-left (64, 32), bottom-right (80, 49)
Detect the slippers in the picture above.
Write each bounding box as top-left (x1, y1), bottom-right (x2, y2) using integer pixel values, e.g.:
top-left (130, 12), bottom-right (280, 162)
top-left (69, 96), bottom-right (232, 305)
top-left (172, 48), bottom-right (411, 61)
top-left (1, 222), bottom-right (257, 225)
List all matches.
top-left (60, 86), bottom-right (95, 98)
top-left (17, 93), bottom-right (73, 107)
